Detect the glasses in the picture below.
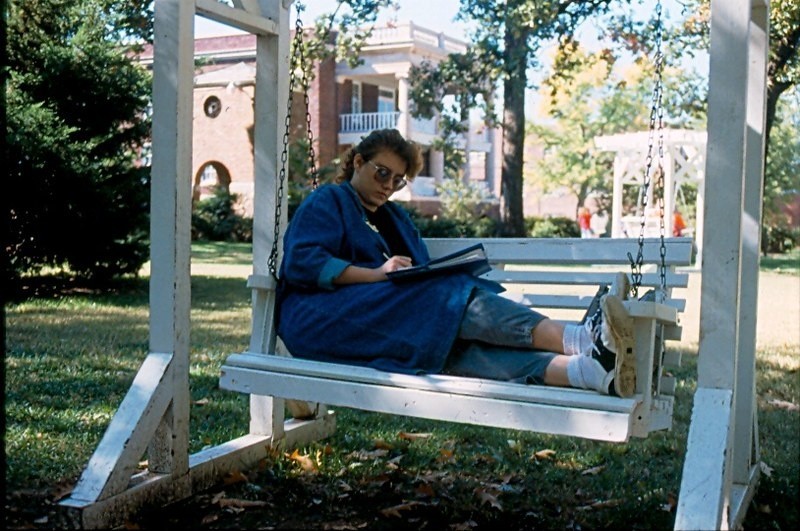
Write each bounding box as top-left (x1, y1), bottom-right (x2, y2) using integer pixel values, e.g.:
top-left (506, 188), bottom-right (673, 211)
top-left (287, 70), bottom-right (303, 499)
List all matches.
top-left (369, 160), bottom-right (407, 191)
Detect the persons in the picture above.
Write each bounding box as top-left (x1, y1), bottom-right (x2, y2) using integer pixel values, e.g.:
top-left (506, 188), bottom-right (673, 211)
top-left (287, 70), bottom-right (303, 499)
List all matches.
top-left (577, 198), bottom-right (608, 238)
top-left (673, 206), bottom-right (685, 237)
top-left (276, 129), bottom-right (637, 398)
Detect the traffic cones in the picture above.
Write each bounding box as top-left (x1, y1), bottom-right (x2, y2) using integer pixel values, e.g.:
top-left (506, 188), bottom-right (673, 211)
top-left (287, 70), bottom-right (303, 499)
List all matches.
top-left (672, 210), bottom-right (686, 239)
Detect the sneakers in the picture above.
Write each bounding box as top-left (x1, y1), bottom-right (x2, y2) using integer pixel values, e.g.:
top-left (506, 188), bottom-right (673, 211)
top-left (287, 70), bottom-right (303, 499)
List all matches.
top-left (589, 293), bottom-right (637, 398)
top-left (578, 271), bottom-right (630, 356)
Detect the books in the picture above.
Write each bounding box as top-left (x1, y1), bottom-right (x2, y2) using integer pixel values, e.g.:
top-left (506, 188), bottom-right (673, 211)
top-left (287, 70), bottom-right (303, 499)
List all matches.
top-left (384, 242), bottom-right (506, 295)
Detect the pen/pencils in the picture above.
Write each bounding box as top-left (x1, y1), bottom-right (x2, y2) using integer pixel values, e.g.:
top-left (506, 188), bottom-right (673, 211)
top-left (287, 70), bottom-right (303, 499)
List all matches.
top-left (383, 253), bottom-right (390, 260)
